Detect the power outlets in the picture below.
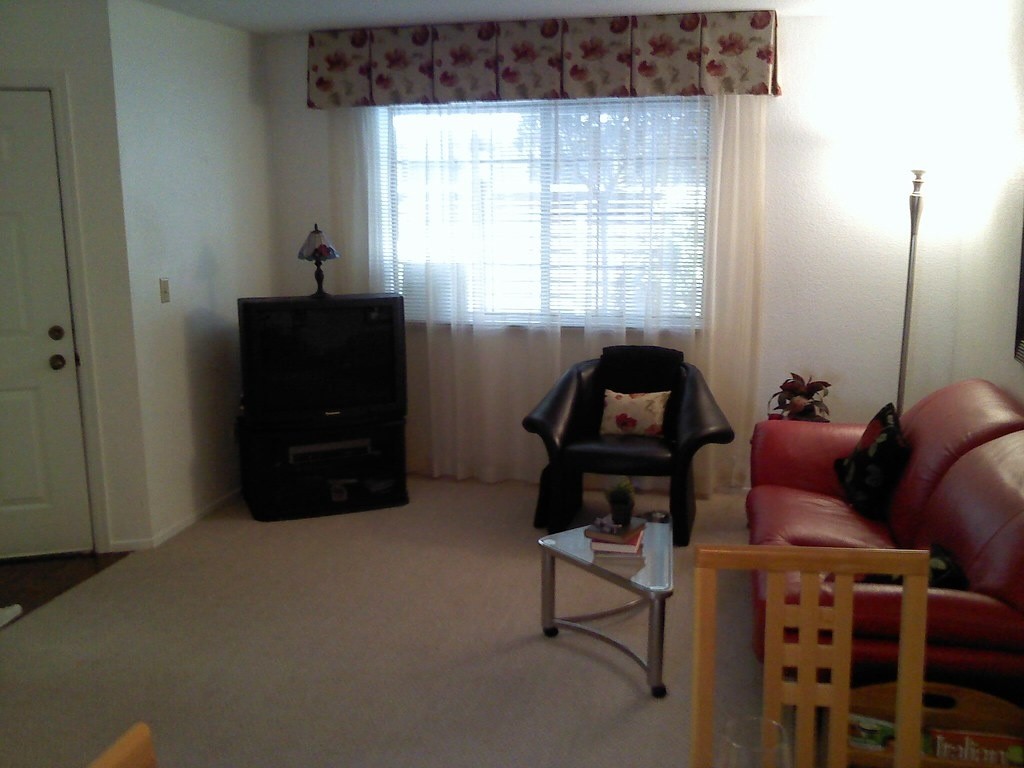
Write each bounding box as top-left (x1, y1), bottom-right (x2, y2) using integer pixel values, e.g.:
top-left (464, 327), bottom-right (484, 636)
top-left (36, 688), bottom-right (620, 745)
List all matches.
top-left (160, 278), bottom-right (171, 303)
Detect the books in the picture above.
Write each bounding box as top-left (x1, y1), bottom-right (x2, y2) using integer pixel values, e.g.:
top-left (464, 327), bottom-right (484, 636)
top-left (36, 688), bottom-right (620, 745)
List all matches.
top-left (583, 513), bottom-right (647, 558)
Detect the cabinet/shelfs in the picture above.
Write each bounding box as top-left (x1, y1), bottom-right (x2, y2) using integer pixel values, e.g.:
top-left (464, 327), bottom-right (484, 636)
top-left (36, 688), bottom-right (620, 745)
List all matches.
top-left (232, 417), bottom-right (410, 521)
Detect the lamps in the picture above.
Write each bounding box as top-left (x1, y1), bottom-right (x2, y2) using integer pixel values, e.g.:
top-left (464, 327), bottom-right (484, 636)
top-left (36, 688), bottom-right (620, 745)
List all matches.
top-left (298, 223), bottom-right (341, 298)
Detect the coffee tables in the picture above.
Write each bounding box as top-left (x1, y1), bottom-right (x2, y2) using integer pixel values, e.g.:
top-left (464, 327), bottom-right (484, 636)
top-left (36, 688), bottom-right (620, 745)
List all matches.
top-left (534, 519), bottom-right (673, 698)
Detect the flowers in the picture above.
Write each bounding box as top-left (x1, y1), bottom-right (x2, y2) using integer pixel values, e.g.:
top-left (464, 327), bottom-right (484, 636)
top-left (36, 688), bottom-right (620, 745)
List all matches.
top-left (767, 372), bottom-right (832, 423)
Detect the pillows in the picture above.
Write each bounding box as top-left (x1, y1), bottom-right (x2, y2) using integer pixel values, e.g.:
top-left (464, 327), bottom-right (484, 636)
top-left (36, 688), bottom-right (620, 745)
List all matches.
top-left (834, 403), bottom-right (914, 522)
top-left (599, 389), bottom-right (671, 440)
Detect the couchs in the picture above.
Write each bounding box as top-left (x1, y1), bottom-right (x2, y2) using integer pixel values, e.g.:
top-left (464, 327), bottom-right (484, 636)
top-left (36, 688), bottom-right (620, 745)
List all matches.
top-left (521, 346), bottom-right (734, 546)
top-left (744, 377), bottom-right (1024, 768)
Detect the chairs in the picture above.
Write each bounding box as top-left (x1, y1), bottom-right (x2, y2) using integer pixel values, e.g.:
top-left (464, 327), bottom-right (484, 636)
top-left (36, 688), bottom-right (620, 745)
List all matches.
top-left (689, 546), bottom-right (929, 768)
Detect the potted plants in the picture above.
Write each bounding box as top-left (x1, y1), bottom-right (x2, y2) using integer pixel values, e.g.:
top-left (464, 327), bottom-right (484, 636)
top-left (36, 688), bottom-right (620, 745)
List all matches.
top-left (604, 474), bottom-right (636, 525)
